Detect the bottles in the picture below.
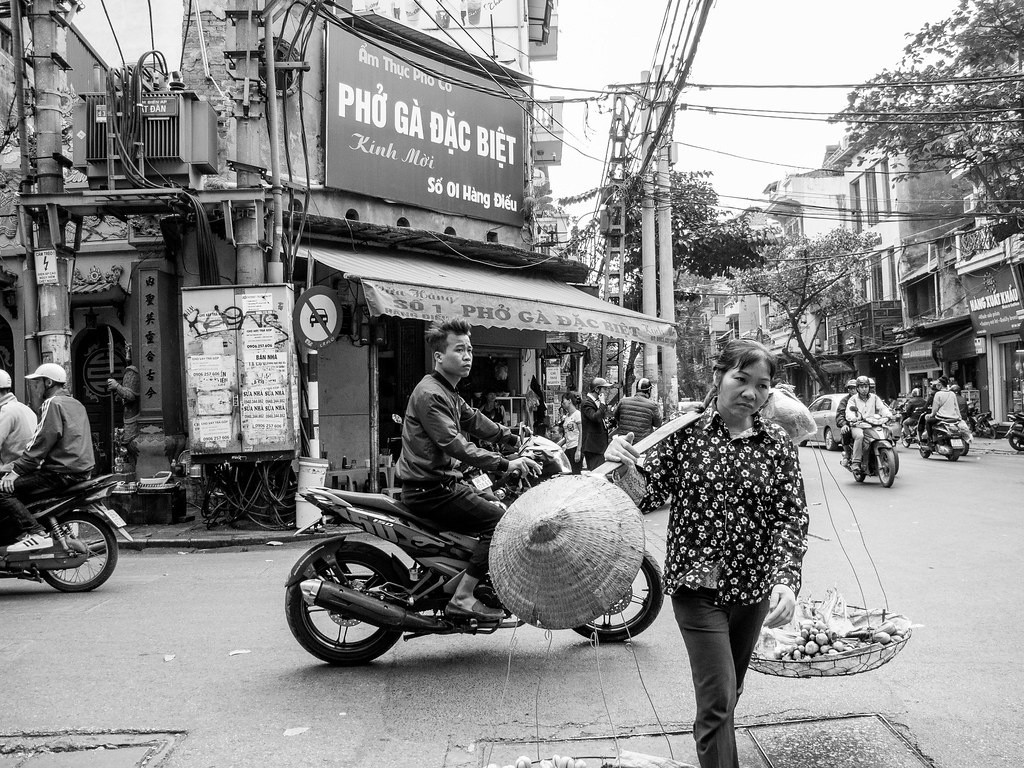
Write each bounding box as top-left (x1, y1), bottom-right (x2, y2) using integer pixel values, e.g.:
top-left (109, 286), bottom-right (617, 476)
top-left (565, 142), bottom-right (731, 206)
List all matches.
top-left (342, 455), bottom-right (348, 469)
top-left (385, 457), bottom-right (391, 467)
top-left (352, 460), bottom-right (357, 469)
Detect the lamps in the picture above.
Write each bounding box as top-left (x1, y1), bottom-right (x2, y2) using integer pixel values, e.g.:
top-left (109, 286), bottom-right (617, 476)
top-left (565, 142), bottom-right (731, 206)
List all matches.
top-left (965, 248), bottom-right (991, 262)
top-left (945, 244), bottom-right (962, 253)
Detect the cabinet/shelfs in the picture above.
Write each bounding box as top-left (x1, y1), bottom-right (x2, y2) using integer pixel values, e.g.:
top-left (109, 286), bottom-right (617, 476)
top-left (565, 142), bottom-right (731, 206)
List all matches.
top-left (960, 390), bottom-right (980, 413)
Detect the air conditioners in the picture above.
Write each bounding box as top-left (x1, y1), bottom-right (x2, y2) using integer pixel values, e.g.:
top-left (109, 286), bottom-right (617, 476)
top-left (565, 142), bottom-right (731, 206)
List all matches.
top-left (963, 193), bottom-right (981, 214)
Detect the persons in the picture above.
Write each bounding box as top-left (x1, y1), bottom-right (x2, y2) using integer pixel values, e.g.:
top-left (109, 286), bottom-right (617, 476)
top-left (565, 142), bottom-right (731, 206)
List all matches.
top-left (614, 378), bottom-right (663, 445)
top-left (107, 340), bottom-right (140, 437)
top-left (395, 314), bottom-right (543, 622)
top-left (903, 388), bottom-right (926, 440)
top-left (949, 385), bottom-right (968, 420)
top-left (0, 363), bottom-right (96, 551)
top-left (603, 339), bottom-right (809, 768)
top-left (0, 368), bottom-right (38, 471)
top-left (581, 378), bottom-right (613, 472)
top-left (836, 376), bottom-right (893, 470)
top-left (916, 375), bottom-right (961, 447)
top-left (532, 392), bottom-right (585, 475)
top-left (480, 388), bottom-right (507, 427)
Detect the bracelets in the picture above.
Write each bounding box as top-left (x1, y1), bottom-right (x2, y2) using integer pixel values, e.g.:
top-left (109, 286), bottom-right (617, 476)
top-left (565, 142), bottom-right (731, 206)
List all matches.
top-left (11, 470), bottom-right (20, 476)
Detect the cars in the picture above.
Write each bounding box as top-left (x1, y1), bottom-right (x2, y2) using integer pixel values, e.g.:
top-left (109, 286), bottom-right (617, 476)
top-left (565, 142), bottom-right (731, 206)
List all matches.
top-left (796, 393), bottom-right (902, 451)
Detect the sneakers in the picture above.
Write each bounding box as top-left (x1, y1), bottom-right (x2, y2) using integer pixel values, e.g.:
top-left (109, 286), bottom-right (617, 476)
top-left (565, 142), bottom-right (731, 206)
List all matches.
top-left (7, 534), bottom-right (54, 552)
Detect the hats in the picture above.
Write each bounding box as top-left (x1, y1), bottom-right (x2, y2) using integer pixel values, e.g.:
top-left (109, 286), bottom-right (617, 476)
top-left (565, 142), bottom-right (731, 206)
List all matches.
top-left (489, 473), bottom-right (645, 630)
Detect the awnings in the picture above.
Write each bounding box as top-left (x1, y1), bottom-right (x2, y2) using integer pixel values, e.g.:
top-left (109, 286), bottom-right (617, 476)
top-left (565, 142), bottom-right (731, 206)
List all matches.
top-left (279, 245), bottom-right (679, 348)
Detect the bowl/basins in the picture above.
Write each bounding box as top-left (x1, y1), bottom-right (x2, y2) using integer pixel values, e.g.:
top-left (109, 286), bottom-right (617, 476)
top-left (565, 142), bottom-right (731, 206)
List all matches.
top-left (496, 393), bottom-right (510, 397)
top-left (474, 392), bottom-right (483, 398)
top-left (345, 465), bottom-right (352, 468)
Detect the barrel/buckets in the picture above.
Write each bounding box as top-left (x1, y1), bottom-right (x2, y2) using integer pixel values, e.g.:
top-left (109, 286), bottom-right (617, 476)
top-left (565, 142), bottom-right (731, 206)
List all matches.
top-left (295, 491), bottom-right (328, 528)
top-left (297, 456), bottom-right (329, 493)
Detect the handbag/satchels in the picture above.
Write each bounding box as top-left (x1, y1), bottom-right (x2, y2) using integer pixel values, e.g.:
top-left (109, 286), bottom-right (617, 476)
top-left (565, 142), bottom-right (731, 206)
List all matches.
top-left (760, 389), bottom-right (818, 446)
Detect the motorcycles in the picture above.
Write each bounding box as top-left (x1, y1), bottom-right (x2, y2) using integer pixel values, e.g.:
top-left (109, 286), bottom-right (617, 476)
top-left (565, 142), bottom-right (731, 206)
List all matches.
top-left (1003, 411), bottom-right (1024, 454)
top-left (965, 398), bottom-right (997, 440)
top-left (283, 421), bottom-right (665, 667)
top-left (886, 393), bottom-right (974, 462)
top-left (0, 473), bottom-right (134, 593)
top-left (839, 406), bottom-right (903, 488)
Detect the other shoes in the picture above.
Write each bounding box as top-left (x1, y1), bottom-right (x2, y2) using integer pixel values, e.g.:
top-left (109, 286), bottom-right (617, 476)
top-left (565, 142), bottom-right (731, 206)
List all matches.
top-left (842, 457), bottom-right (848, 466)
top-left (905, 436), bottom-right (911, 439)
top-left (445, 600), bottom-right (504, 622)
top-left (850, 463), bottom-right (861, 471)
top-left (926, 440), bottom-right (933, 446)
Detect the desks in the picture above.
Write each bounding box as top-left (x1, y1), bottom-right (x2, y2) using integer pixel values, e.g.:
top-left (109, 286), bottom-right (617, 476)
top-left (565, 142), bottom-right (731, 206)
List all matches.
top-left (327, 466), bottom-right (388, 492)
top-left (387, 468), bottom-right (396, 488)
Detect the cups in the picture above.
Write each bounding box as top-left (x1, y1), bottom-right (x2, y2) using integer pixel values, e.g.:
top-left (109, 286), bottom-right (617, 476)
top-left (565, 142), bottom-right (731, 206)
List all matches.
top-left (365, 459), bottom-right (370, 468)
top-left (512, 389), bottom-right (516, 396)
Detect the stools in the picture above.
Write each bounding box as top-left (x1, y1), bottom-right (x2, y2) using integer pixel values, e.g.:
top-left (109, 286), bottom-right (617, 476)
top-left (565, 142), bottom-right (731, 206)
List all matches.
top-left (341, 479), bottom-right (370, 491)
top-left (381, 488), bottom-right (402, 498)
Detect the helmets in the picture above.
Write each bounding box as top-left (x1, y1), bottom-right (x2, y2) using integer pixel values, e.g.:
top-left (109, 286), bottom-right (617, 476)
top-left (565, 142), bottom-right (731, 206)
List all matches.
top-left (25, 363), bottom-right (66, 383)
top-left (912, 388), bottom-right (921, 396)
top-left (846, 379), bottom-right (857, 387)
top-left (930, 380), bottom-right (938, 390)
top-left (0, 369), bottom-right (10, 388)
top-left (636, 378), bottom-right (652, 390)
top-left (857, 375), bottom-right (870, 386)
top-left (951, 385), bottom-right (960, 394)
top-left (590, 377), bottom-right (613, 392)
